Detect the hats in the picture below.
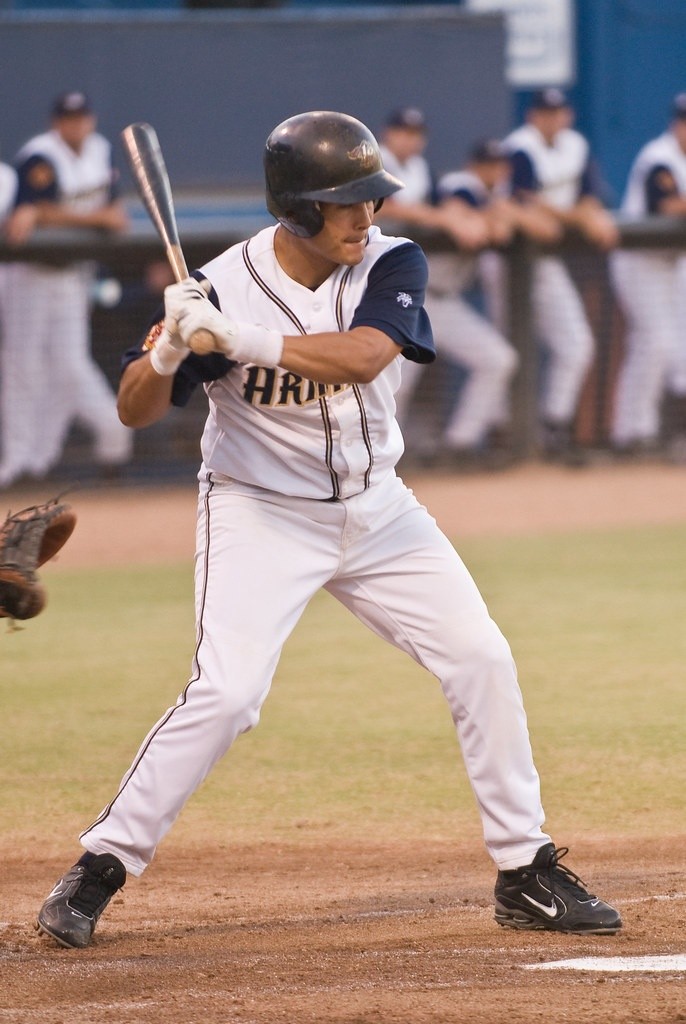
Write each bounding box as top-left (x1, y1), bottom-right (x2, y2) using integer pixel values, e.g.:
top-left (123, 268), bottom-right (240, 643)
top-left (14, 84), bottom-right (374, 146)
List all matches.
top-left (54, 93), bottom-right (93, 118)
top-left (532, 89), bottom-right (567, 108)
top-left (387, 106), bottom-right (425, 133)
top-left (675, 94), bottom-right (686, 120)
top-left (476, 139), bottom-right (513, 161)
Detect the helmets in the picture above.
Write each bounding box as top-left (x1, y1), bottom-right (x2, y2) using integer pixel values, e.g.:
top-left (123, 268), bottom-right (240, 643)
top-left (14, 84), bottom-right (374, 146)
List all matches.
top-left (264, 111), bottom-right (403, 238)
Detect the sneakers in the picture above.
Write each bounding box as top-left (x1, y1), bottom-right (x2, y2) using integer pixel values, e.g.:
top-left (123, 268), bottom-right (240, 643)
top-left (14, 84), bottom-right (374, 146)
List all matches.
top-left (37, 849), bottom-right (126, 949)
top-left (493, 842), bottom-right (623, 933)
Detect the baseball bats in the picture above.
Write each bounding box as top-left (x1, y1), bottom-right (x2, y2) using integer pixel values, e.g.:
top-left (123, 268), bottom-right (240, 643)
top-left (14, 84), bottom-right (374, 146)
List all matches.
top-left (115, 117), bottom-right (218, 359)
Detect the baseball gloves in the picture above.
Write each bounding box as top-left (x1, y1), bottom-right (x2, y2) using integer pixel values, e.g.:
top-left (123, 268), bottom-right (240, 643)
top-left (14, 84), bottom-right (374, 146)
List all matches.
top-left (0, 506), bottom-right (78, 622)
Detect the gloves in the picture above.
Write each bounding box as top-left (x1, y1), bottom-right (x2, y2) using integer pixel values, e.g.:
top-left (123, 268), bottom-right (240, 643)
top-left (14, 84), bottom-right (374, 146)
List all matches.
top-left (150, 278), bottom-right (282, 376)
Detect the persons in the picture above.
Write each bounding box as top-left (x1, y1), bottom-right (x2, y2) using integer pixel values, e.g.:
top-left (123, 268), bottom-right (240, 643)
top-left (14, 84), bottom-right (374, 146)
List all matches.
top-left (359, 86), bottom-right (686, 469)
top-left (1, 90), bottom-right (172, 502)
top-left (32, 111), bottom-right (624, 952)
